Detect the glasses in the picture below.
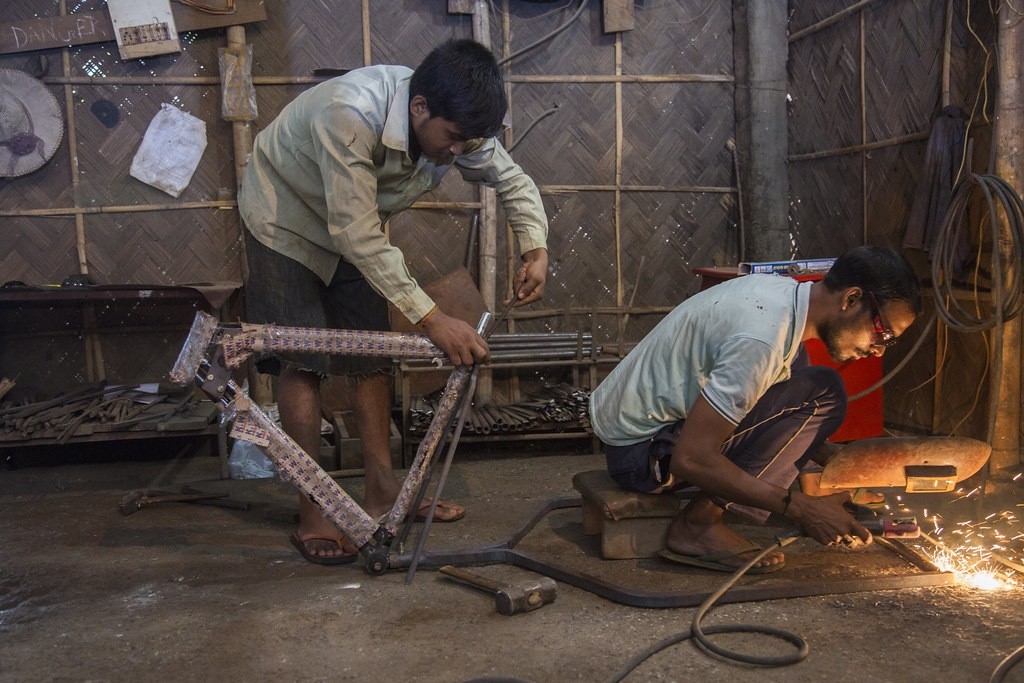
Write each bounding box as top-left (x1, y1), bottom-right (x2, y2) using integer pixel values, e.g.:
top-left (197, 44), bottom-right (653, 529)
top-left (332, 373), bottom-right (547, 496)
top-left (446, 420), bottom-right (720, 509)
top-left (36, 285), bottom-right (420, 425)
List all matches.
top-left (865, 290), bottom-right (900, 348)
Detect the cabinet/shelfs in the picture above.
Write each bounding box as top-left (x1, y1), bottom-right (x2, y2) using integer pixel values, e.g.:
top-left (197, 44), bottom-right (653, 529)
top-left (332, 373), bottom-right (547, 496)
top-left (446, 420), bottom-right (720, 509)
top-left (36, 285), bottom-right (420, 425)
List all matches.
top-left (0, 280), bottom-right (245, 479)
top-left (392, 353), bottom-right (640, 469)
top-left (696, 266), bottom-right (883, 444)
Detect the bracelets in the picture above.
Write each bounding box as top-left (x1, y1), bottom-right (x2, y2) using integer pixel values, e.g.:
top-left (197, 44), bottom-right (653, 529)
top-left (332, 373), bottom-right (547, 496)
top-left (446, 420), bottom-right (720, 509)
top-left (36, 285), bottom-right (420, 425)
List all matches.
top-left (421, 305), bottom-right (439, 326)
top-left (782, 487), bottom-right (792, 516)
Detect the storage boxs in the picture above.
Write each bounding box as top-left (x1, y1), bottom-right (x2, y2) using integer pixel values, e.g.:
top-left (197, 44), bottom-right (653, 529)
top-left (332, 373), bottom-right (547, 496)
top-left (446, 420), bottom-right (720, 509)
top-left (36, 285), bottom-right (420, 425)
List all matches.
top-left (334, 410), bottom-right (402, 470)
top-left (737, 257), bottom-right (838, 275)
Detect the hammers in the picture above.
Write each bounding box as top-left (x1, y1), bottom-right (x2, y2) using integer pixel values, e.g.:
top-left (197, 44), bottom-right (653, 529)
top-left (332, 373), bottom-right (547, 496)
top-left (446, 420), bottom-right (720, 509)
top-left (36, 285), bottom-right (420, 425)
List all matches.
top-left (437, 564), bottom-right (558, 616)
top-left (121, 490), bottom-right (228, 520)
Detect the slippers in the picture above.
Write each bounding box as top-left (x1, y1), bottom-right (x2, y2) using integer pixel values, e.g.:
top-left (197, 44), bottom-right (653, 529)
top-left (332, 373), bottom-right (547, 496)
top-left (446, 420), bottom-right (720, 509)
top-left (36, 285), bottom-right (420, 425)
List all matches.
top-left (415, 497), bottom-right (466, 523)
top-left (659, 537), bottom-right (786, 574)
top-left (290, 531), bottom-right (359, 565)
top-left (852, 488), bottom-right (887, 508)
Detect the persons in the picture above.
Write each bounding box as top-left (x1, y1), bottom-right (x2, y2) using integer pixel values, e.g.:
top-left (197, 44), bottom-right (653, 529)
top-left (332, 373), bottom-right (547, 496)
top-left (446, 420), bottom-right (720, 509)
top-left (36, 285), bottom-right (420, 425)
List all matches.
top-left (236, 39), bottom-right (549, 566)
top-left (589, 246), bottom-right (921, 573)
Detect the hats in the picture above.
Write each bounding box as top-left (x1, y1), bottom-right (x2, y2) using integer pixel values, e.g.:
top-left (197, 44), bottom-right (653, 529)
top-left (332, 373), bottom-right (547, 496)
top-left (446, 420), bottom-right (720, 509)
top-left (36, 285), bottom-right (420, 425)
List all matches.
top-left (0, 69), bottom-right (64, 177)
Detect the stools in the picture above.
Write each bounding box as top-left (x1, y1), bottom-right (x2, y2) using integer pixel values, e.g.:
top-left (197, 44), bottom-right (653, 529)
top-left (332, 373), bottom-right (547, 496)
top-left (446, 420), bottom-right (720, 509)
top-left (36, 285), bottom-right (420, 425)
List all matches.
top-left (572, 467), bottom-right (682, 559)
